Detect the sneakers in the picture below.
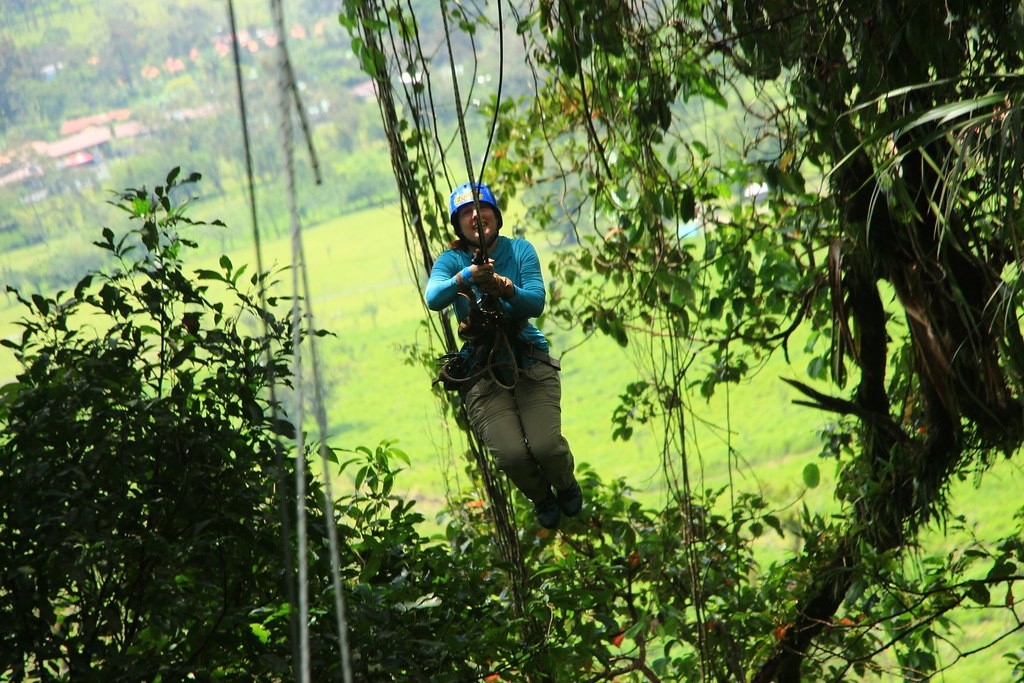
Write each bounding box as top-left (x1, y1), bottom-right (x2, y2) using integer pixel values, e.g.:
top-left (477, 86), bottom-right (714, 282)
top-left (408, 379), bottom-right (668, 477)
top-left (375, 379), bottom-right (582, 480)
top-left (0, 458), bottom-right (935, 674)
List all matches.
top-left (558, 479), bottom-right (583, 517)
top-left (533, 489), bottom-right (561, 528)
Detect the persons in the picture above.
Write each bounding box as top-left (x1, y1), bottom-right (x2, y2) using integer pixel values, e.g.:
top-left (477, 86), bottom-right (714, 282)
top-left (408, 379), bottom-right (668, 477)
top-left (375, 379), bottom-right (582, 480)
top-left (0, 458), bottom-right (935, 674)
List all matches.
top-left (425, 180), bottom-right (584, 530)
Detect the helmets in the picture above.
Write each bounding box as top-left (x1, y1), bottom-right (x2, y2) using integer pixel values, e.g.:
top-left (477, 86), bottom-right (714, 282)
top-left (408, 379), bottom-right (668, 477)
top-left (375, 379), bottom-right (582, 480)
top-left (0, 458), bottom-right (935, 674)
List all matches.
top-left (449, 182), bottom-right (502, 235)
